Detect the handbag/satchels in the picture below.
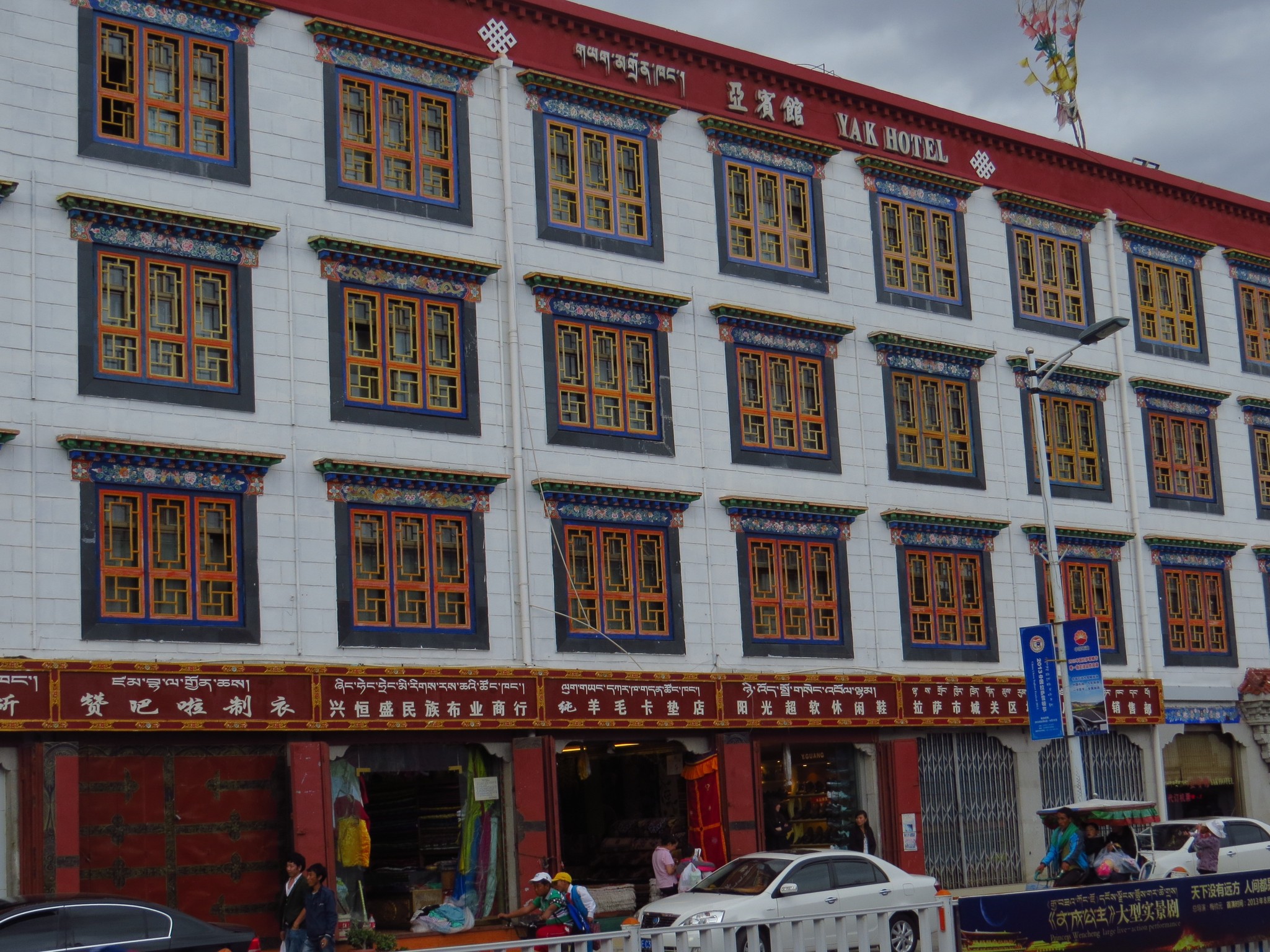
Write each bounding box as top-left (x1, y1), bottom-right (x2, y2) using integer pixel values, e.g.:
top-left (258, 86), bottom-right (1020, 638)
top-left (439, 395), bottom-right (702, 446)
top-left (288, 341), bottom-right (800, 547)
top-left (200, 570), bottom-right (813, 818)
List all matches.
top-left (1090, 843), bottom-right (1141, 880)
top-left (678, 863), bottom-right (701, 893)
top-left (587, 921), bottom-right (601, 951)
top-left (280, 940), bottom-right (286, 952)
top-left (549, 898), bottom-right (590, 932)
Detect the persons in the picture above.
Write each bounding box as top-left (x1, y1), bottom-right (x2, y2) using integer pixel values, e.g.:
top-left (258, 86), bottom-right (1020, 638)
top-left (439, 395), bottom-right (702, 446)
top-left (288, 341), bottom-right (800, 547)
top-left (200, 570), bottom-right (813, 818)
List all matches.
top-left (280, 852), bottom-right (313, 952)
top-left (764, 797), bottom-right (792, 850)
top-left (1183, 819), bottom-right (1226, 875)
top-left (1035, 807), bottom-right (1136, 888)
top-left (552, 872), bottom-right (596, 952)
top-left (300, 863), bottom-right (338, 952)
top-left (676, 844), bottom-right (715, 882)
top-left (652, 835), bottom-right (679, 897)
top-left (497, 872), bottom-right (574, 952)
top-left (848, 810), bottom-right (876, 855)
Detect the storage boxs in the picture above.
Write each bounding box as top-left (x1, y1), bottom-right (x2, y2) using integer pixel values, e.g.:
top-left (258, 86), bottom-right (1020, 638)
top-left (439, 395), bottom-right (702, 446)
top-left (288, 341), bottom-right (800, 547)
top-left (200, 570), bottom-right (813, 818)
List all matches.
top-left (408, 889), bottom-right (442, 918)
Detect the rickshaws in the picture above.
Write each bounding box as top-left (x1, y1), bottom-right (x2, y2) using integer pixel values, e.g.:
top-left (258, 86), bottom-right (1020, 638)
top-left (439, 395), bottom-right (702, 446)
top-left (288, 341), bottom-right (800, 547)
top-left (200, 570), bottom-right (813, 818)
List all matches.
top-left (1026, 792), bottom-right (1161, 892)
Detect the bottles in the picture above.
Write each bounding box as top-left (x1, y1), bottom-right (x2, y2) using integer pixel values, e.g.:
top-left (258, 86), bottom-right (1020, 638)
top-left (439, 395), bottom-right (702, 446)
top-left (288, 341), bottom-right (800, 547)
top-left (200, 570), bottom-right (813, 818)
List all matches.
top-left (368, 914), bottom-right (375, 930)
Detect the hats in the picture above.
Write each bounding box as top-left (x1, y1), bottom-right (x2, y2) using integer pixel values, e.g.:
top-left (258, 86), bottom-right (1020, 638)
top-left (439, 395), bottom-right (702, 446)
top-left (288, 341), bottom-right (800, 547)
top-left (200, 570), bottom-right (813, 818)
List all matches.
top-left (1205, 819), bottom-right (1226, 838)
top-left (529, 872), bottom-right (552, 884)
top-left (552, 872), bottom-right (571, 883)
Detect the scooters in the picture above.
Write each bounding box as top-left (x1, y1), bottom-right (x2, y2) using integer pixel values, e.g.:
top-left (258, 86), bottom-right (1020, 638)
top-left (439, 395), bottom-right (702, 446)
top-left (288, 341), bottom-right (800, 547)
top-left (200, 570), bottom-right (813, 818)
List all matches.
top-left (499, 916), bottom-right (600, 952)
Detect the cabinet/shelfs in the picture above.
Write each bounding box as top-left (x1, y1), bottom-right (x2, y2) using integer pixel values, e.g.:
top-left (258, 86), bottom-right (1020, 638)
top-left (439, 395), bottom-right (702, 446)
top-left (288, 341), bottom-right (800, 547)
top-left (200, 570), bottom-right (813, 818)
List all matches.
top-left (788, 793), bottom-right (834, 848)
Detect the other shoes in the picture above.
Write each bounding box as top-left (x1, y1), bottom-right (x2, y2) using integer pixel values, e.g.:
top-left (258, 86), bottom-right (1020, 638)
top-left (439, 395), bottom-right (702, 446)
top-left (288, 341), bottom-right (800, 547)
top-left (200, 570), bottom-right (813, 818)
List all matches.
top-left (763, 789), bottom-right (788, 803)
top-left (796, 778), bottom-right (848, 798)
top-left (792, 800), bottom-right (847, 820)
top-left (794, 825), bottom-right (849, 850)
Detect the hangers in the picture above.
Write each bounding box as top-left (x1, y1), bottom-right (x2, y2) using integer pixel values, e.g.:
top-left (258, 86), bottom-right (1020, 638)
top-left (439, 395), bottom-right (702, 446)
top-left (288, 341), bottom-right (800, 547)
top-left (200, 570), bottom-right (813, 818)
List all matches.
top-left (351, 815), bottom-right (359, 825)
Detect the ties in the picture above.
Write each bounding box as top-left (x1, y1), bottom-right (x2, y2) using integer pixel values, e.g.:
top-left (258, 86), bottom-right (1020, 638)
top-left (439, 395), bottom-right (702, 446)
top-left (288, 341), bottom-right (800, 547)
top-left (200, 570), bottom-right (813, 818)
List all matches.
top-left (566, 893), bottom-right (574, 904)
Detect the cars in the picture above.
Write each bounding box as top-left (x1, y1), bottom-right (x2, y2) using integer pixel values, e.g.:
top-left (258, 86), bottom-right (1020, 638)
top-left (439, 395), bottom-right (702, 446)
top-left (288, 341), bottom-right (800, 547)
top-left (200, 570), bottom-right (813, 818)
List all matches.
top-left (1137, 815), bottom-right (1270, 880)
top-left (0, 889), bottom-right (262, 952)
top-left (631, 847), bottom-right (943, 952)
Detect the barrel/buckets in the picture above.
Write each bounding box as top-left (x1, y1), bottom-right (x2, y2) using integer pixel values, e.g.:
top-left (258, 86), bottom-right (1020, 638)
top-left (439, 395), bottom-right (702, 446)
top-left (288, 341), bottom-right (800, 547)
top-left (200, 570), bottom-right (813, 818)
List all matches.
top-left (338, 914), bottom-right (351, 940)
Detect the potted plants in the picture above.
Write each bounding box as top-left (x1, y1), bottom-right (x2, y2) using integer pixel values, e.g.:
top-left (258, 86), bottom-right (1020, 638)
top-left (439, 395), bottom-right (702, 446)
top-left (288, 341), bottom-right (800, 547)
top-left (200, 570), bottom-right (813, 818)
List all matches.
top-left (345, 928), bottom-right (376, 952)
